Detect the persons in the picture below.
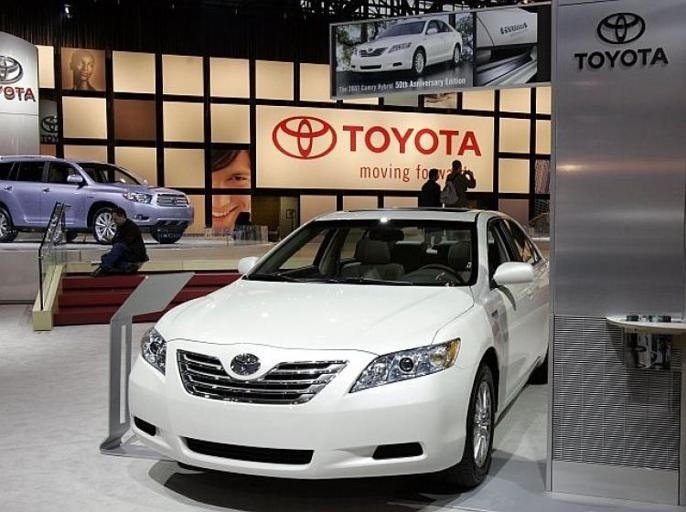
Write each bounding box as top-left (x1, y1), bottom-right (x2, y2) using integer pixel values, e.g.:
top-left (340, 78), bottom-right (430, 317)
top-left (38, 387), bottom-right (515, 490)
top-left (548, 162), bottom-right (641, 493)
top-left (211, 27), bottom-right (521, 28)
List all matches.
top-left (69, 50), bottom-right (95, 90)
top-left (212, 150), bottom-right (251, 233)
top-left (445, 160), bottom-right (476, 207)
top-left (422, 169), bottom-right (442, 207)
top-left (112, 208), bottom-right (149, 263)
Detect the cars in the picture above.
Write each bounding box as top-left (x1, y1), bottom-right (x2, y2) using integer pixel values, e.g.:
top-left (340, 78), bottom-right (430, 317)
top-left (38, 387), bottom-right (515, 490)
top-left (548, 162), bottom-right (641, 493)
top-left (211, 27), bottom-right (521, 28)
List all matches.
top-left (124, 205), bottom-right (551, 500)
top-left (345, 16), bottom-right (464, 81)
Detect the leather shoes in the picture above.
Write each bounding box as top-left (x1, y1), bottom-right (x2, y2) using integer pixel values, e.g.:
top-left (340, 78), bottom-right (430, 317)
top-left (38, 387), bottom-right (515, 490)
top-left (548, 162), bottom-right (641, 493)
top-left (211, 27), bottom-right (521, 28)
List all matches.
top-left (90, 267), bottom-right (109, 277)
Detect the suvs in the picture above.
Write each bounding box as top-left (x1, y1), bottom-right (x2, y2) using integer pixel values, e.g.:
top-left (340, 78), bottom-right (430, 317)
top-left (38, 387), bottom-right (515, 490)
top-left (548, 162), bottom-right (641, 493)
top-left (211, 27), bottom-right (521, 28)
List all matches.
top-left (0, 152), bottom-right (195, 243)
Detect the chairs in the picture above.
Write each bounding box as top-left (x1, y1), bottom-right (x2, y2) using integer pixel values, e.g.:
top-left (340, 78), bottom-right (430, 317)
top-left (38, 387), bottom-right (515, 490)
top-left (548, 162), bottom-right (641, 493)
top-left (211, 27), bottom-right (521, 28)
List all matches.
top-left (342, 228), bottom-right (472, 284)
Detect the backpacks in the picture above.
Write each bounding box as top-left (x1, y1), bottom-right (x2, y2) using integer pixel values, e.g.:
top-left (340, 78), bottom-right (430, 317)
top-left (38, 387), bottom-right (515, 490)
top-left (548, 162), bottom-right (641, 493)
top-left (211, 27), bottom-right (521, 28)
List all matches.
top-left (440, 173), bottom-right (465, 204)
